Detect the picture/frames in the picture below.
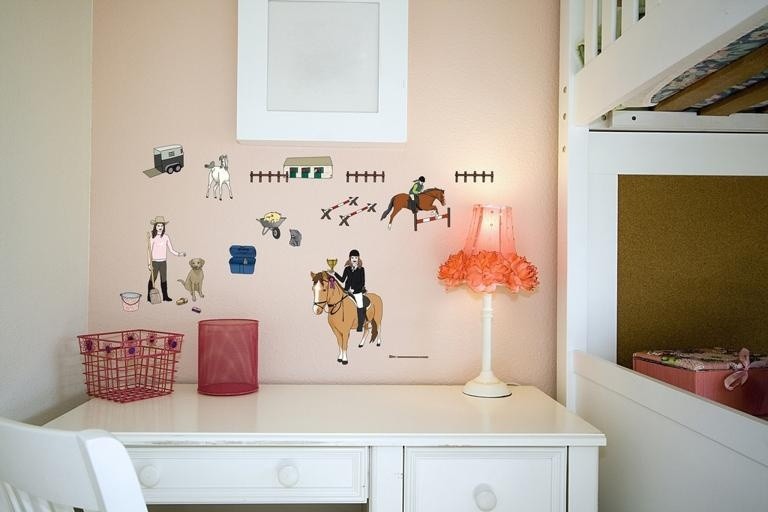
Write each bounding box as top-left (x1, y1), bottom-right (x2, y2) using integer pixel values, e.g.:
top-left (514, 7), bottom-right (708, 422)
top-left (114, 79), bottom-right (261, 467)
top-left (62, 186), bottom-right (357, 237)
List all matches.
top-left (235, 1), bottom-right (408, 144)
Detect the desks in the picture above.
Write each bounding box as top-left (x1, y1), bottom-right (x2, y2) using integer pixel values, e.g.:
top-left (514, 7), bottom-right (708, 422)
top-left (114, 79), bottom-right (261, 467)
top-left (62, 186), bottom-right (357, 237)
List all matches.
top-left (41, 379), bottom-right (606, 512)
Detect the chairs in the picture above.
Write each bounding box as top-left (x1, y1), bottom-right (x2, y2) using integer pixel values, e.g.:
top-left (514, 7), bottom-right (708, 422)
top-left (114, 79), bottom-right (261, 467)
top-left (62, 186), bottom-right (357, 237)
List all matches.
top-left (1, 411), bottom-right (151, 512)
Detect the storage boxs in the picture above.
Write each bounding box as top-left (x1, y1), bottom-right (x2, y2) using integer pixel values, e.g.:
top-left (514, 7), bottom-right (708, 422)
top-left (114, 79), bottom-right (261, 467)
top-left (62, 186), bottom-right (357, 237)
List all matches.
top-left (632, 338), bottom-right (766, 414)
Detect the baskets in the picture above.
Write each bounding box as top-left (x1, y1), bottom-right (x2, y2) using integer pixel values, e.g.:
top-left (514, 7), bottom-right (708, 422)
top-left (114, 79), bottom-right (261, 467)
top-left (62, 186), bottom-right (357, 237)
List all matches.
top-left (79, 331), bottom-right (185, 403)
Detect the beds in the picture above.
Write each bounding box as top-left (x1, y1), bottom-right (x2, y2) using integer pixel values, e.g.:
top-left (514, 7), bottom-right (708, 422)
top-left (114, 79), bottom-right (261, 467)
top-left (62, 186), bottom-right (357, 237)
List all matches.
top-left (550, 207), bottom-right (767, 512)
top-left (545, 0), bottom-right (767, 205)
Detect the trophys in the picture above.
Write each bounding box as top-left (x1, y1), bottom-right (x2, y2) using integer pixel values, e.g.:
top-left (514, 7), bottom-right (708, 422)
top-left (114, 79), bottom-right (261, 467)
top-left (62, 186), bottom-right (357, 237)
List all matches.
top-left (327, 257), bottom-right (338, 273)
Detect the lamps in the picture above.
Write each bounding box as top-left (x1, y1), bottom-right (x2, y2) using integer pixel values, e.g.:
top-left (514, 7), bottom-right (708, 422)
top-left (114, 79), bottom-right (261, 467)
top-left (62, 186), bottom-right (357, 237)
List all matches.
top-left (437, 203), bottom-right (542, 400)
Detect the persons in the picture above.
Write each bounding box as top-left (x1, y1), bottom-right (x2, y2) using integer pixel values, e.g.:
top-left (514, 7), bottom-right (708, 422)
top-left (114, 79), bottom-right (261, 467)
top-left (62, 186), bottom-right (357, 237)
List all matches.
top-left (409, 176), bottom-right (425, 214)
top-left (327, 249), bottom-right (366, 332)
top-left (147, 217), bottom-right (186, 304)
